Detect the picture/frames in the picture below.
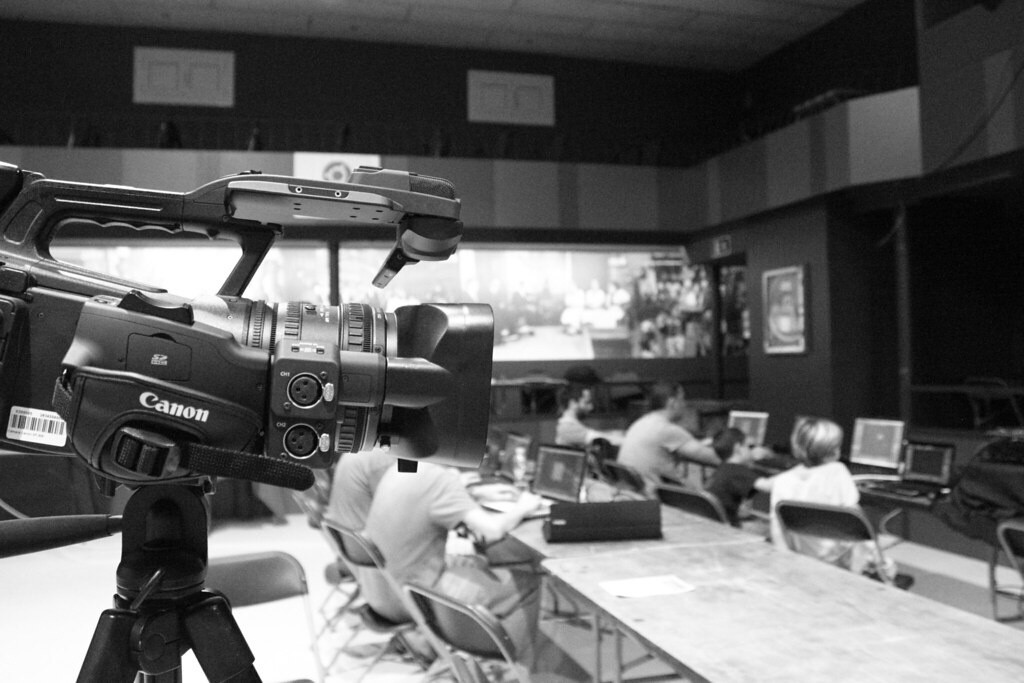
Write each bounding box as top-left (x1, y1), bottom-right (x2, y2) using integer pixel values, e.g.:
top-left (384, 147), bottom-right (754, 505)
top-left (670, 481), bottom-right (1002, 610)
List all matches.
top-left (759, 263), bottom-right (805, 352)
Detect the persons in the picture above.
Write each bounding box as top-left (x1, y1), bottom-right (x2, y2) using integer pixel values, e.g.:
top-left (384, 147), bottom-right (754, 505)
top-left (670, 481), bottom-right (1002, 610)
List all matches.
top-left (555, 385), bottom-right (625, 449)
top-left (615, 380), bottom-right (723, 500)
top-left (770, 416), bottom-right (900, 581)
top-left (704, 428), bottom-right (774, 540)
top-left (326, 435), bottom-right (541, 676)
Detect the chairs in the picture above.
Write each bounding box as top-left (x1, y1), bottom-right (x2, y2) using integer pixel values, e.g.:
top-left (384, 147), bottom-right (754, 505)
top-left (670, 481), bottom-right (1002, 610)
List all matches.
top-left (770, 501), bottom-right (915, 587)
top-left (311, 523), bottom-right (408, 668)
top-left (293, 466), bottom-right (359, 632)
top-left (655, 481), bottom-right (733, 529)
top-left (595, 459), bottom-right (647, 491)
top-left (401, 587), bottom-right (520, 683)
top-left (972, 516), bottom-right (1023, 622)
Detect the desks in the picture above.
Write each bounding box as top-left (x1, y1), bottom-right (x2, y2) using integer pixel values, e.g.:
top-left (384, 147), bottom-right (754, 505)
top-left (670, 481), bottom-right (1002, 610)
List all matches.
top-left (467, 396), bottom-right (1024, 683)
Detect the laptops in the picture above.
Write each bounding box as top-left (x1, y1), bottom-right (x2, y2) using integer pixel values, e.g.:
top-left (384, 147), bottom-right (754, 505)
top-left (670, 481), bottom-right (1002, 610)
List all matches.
top-left (868, 441), bottom-right (956, 497)
top-left (465, 430), bottom-right (587, 521)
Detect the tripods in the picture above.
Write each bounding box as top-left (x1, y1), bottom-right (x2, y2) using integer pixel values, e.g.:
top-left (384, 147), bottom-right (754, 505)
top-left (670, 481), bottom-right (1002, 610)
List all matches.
top-left (74, 468), bottom-right (264, 683)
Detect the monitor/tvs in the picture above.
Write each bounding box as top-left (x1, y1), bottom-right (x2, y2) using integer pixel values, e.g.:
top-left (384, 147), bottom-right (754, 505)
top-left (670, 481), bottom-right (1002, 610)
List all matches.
top-left (848, 417), bottom-right (904, 474)
top-left (728, 410), bottom-right (769, 445)
